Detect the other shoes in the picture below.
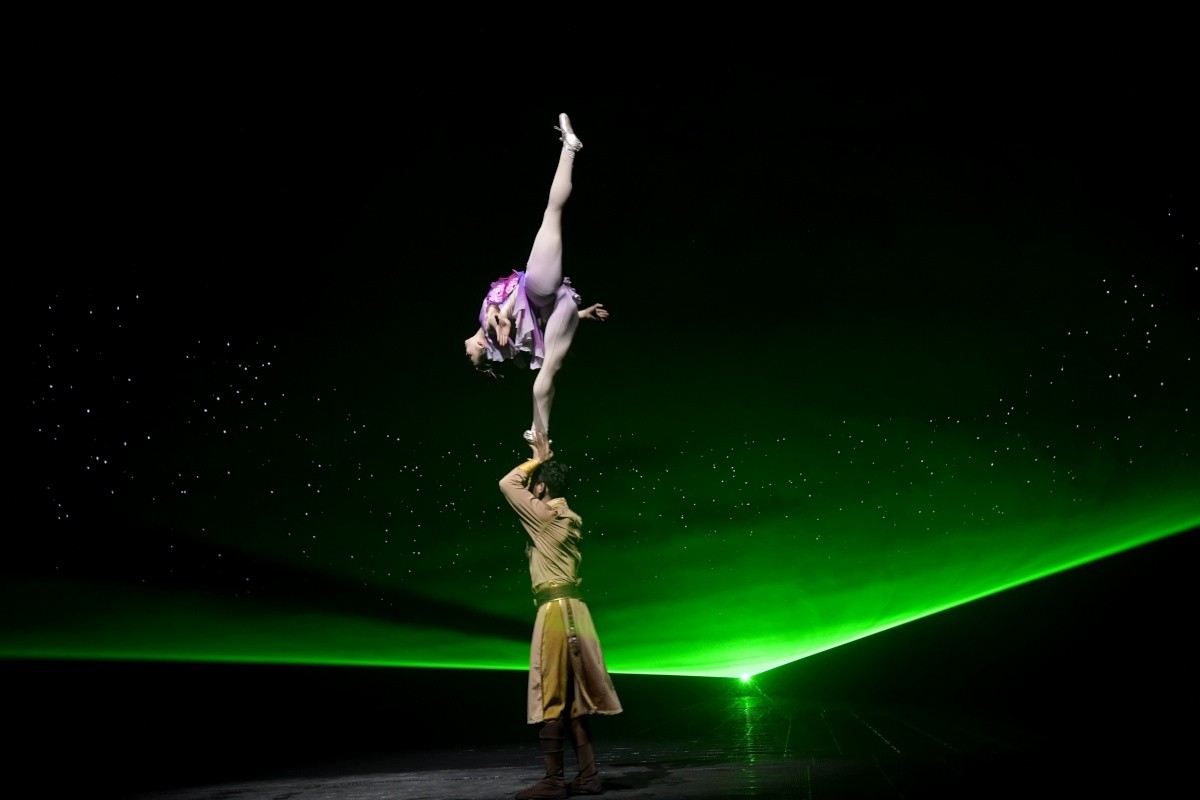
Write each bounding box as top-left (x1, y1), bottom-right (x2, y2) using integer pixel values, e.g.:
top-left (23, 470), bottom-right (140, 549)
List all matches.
top-left (524, 431), bottom-right (549, 447)
top-left (553, 112), bottom-right (583, 151)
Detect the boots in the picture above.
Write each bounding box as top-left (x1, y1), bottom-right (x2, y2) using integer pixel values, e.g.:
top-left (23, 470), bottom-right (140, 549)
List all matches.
top-left (566, 742), bottom-right (601, 793)
top-left (515, 726), bottom-right (567, 798)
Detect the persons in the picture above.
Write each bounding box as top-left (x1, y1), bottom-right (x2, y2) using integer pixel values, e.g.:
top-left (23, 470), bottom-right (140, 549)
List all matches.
top-left (499, 432), bottom-right (624, 800)
top-left (464, 113), bottom-right (609, 445)
